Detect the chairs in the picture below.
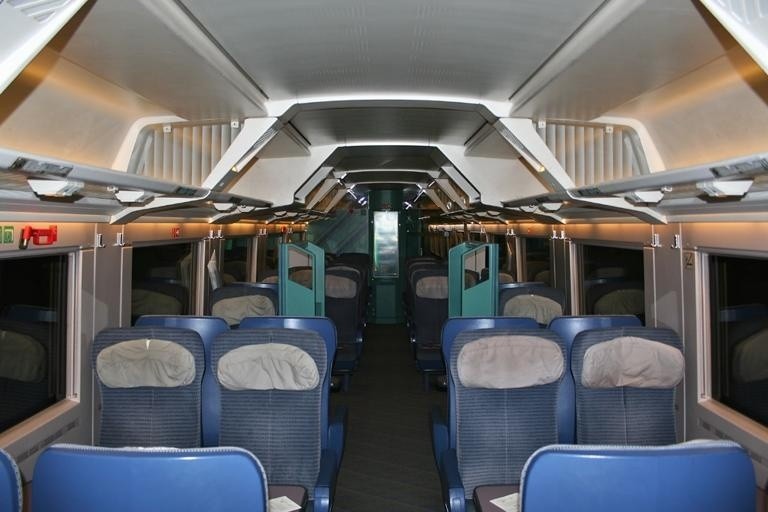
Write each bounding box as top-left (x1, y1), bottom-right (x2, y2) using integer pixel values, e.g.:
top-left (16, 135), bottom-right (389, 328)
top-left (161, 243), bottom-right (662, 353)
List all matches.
top-left (430, 315), bottom-right (757, 510)
top-left (0, 315), bottom-right (345, 511)
top-left (208, 256), bottom-right (372, 390)
top-left (407, 253), bottom-right (565, 407)
